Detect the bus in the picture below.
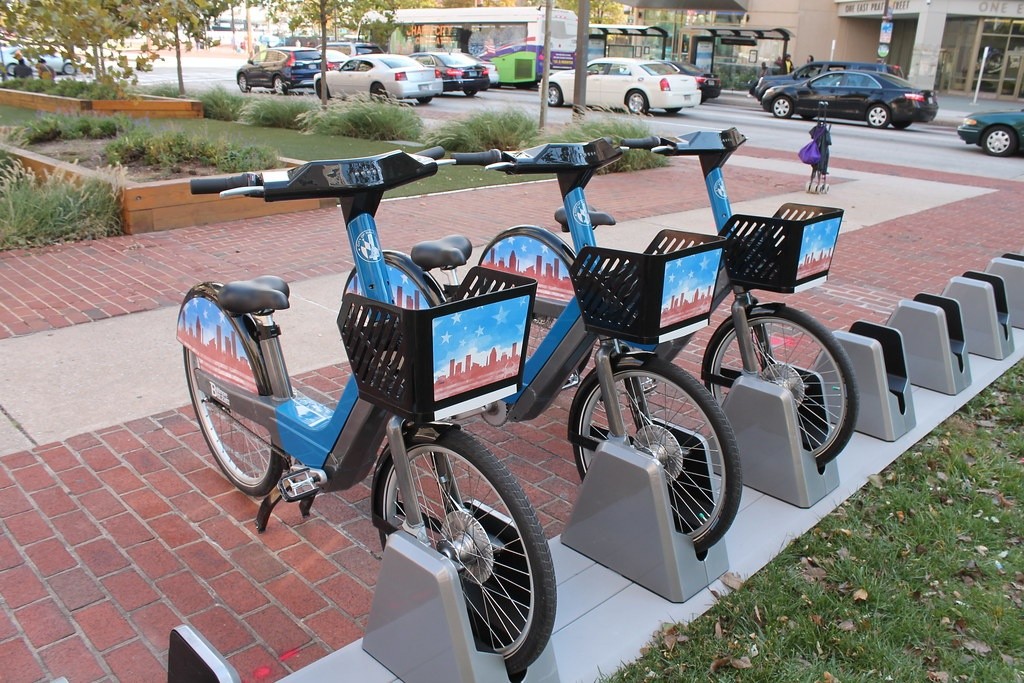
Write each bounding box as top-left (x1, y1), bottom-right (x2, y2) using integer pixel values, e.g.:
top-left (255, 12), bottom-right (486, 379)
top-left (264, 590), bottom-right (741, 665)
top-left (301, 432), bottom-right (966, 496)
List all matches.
top-left (356, 6), bottom-right (577, 90)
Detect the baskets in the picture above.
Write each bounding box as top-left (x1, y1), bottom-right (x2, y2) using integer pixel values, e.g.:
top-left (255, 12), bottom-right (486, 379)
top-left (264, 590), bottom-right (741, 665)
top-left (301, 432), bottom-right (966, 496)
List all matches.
top-left (574, 230), bottom-right (726, 345)
top-left (337, 267), bottom-right (539, 422)
top-left (718, 203), bottom-right (843, 294)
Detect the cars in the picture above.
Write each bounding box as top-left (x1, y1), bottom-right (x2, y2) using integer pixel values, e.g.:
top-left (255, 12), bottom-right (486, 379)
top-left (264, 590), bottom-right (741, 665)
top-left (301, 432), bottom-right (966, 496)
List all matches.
top-left (236, 46), bottom-right (327, 96)
top-left (659, 60), bottom-right (724, 103)
top-left (0, 48), bottom-right (76, 76)
top-left (759, 69), bottom-right (940, 133)
top-left (407, 52), bottom-right (491, 95)
top-left (536, 56), bottom-right (703, 115)
top-left (317, 50), bottom-right (355, 71)
top-left (313, 53), bottom-right (445, 105)
top-left (956, 109), bottom-right (1024, 157)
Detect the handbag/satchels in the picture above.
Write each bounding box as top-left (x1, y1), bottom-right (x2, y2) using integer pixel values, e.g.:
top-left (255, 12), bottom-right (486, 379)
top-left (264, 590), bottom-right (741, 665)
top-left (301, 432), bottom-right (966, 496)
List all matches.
top-left (798, 125), bottom-right (824, 164)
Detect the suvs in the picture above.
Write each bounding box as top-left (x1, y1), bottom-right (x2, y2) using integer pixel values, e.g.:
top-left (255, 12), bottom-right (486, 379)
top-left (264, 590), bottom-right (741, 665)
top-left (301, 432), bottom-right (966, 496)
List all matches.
top-left (315, 41), bottom-right (387, 60)
top-left (753, 61), bottom-right (906, 106)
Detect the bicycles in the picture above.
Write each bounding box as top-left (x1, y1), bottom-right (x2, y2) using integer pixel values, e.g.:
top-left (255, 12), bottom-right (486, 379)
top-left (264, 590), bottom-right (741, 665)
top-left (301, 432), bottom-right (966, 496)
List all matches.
top-left (176, 147), bottom-right (558, 674)
top-left (339, 124), bottom-right (745, 553)
top-left (477, 126), bottom-right (860, 473)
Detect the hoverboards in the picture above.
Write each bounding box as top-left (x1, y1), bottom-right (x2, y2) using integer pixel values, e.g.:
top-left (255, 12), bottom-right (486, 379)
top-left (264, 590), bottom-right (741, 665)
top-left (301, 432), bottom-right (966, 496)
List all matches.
top-left (805, 170), bottom-right (831, 196)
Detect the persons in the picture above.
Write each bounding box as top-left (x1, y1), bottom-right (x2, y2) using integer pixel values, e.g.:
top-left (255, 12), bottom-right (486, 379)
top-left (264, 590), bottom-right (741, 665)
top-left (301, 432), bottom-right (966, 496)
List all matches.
top-left (38, 58), bottom-right (54, 80)
top-left (253, 37), bottom-right (260, 53)
top-left (266, 41), bottom-right (271, 48)
top-left (775, 57), bottom-right (783, 65)
top-left (746, 62), bottom-right (769, 97)
top-left (806, 54), bottom-right (814, 63)
top-left (784, 54), bottom-right (793, 74)
top-left (12, 58), bottom-right (33, 77)
top-left (295, 39), bottom-right (301, 47)
